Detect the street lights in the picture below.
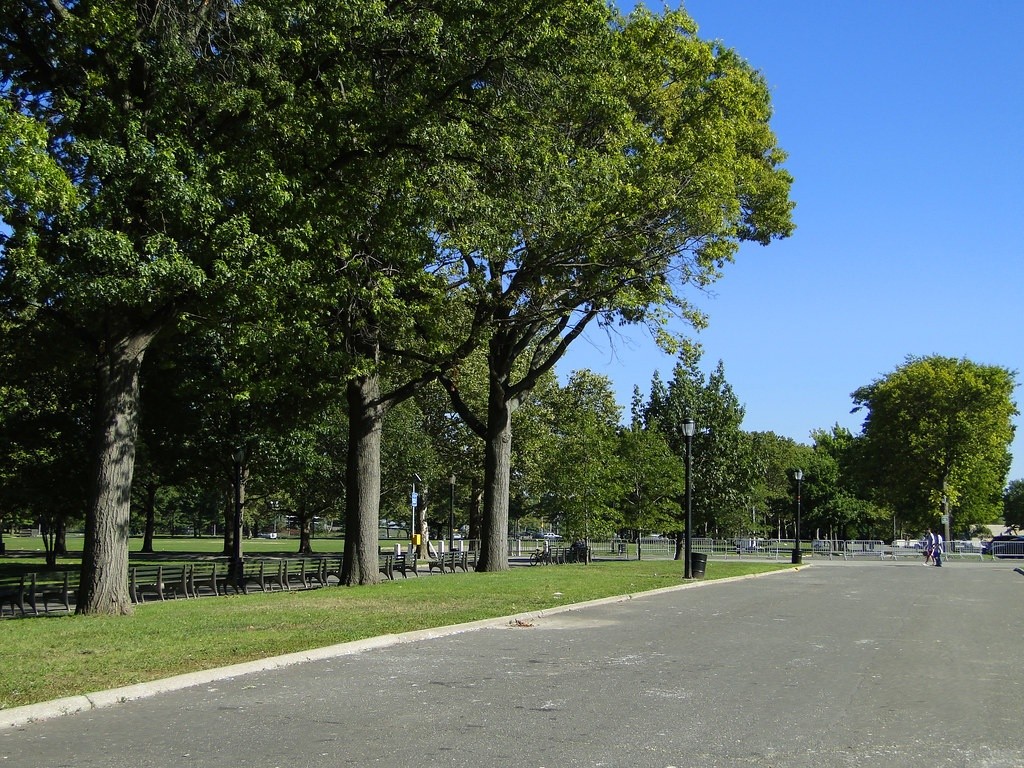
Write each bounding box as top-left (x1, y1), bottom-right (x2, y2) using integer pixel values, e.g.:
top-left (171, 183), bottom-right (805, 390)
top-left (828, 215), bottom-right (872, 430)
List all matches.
top-left (792, 468), bottom-right (803, 564)
top-left (449, 473), bottom-right (456, 552)
top-left (682, 417), bottom-right (696, 578)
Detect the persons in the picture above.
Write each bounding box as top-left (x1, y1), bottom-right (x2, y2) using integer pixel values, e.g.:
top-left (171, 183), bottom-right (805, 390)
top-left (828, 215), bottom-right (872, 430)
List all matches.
top-left (923, 527), bottom-right (943, 567)
top-left (570, 539), bottom-right (586, 561)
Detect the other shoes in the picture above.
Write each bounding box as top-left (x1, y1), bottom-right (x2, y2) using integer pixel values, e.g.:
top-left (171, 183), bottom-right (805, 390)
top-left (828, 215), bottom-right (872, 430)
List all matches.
top-left (925, 561), bottom-right (927, 564)
top-left (935, 564), bottom-right (941, 567)
top-left (930, 564), bottom-right (934, 566)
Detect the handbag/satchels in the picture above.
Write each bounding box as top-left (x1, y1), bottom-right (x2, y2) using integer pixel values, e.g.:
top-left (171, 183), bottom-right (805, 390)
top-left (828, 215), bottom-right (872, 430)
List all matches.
top-left (933, 548), bottom-right (940, 558)
top-left (923, 549), bottom-right (928, 556)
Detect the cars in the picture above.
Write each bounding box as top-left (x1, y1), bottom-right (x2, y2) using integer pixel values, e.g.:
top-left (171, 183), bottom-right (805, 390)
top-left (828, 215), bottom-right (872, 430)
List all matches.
top-left (982, 534), bottom-right (1024, 559)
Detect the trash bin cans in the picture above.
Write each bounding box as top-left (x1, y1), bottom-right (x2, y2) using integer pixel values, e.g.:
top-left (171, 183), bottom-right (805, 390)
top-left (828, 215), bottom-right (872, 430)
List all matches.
top-left (792, 549), bottom-right (802, 564)
top-left (617, 544), bottom-right (626, 553)
top-left (692, 552), bottom-right (707, 580)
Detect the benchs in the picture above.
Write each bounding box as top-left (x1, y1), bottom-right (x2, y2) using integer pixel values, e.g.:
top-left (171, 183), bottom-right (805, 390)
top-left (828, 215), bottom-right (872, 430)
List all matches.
top-left (128, 564), bottom-right (189, 605)
top-left (428, 550), bottom-right (480, 575)
top-left (0, 571), bottom-right (69, 617)
top-left (19, 529), bottom-right (33, 537)
top-left (547, 545), bottom-right (589, 565)
top-left (173, 553), bottom-right (419, 599)
